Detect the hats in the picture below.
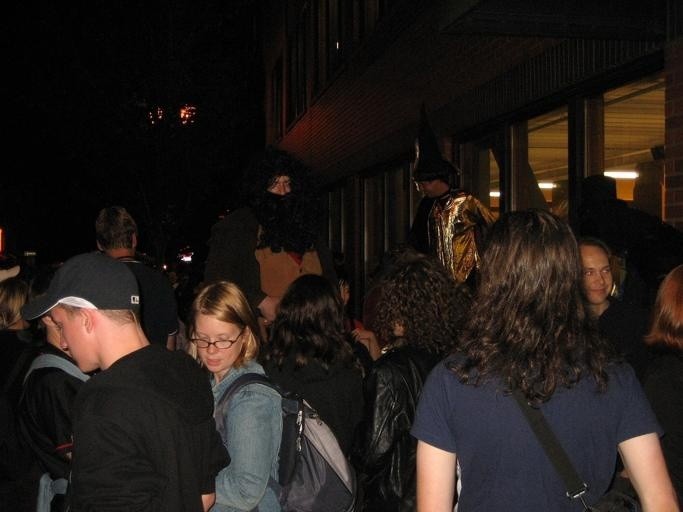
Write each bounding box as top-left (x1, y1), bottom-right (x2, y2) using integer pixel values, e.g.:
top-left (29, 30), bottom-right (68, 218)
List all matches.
top-left (413, 102), bottom-right (451, 181)
top-left (21, 250), bottom-right (140, 321)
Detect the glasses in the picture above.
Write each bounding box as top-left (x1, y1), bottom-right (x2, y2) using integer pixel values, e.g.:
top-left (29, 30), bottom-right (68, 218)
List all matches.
top-left (188, 328), bottom-right (242, 349)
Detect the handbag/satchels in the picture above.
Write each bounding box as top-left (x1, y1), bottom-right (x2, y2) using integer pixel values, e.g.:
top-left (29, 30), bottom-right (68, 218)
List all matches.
top-left (584, 477), bottom-right (642, 512)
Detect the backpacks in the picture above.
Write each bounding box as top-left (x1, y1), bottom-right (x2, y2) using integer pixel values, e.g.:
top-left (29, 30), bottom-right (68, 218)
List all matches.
top-left (215, 373), bottom-right (366, 512)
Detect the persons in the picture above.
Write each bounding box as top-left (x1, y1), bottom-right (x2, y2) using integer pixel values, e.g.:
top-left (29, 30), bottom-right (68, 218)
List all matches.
top-left (407, 208), bottom-right (682, 511)
top-left (199, 147), bottom-right (338, 340)
top-left (574, 174), bottom-right (682, 285)
top-left (0, 249), bottom-right (98, 511)
top-left (18, 250), bottom-right (230, 512)
top-left (93, 203), bottom-right (180, 353)
top-left (572, 235), bottom-right (651, 382)
top-left (187, 268), bottom-right (385, 512)
top-left (613, 262), bottom-right (682, 512)
top-left (352, 253), bottom-right (473, 512)
top-left (411, 159), bottom-right (498, 288)
